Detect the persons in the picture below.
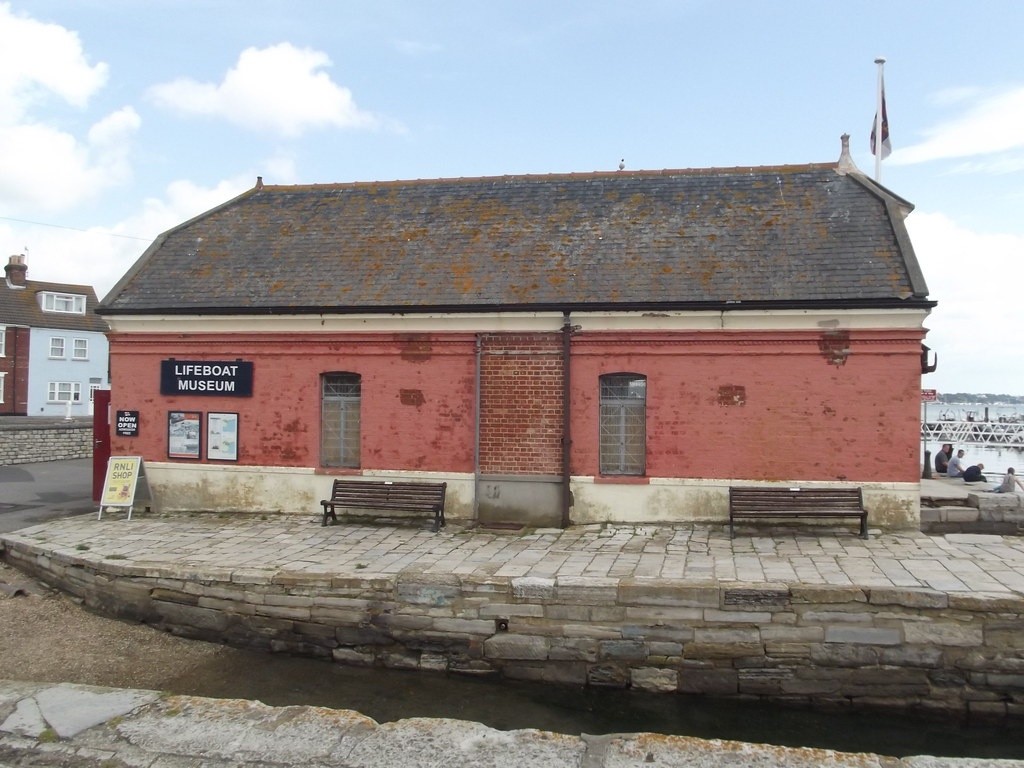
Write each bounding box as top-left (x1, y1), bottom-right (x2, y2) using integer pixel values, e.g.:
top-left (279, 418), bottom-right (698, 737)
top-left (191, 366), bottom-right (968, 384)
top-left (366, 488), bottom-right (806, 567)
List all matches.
top-left (985, 468), bottom-right (1024, 493)
top-left (963, 463), bottom-right (987, 482)
top-left (935, 444), bottom-right (950, 472)
top-left (948, 449), bottom-right (965, 477)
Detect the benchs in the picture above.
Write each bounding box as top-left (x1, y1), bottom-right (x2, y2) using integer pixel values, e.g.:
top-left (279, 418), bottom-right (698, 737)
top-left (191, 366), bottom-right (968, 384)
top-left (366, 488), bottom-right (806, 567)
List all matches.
top-left (321, 479), bottom-right (448, 531)
top-left (729, 487), bottom-right (868, 541)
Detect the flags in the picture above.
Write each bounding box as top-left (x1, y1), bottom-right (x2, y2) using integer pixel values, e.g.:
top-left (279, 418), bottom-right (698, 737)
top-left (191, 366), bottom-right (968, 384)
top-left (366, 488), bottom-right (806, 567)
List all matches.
top-left (871, 68), bottom-right (892, 160)
